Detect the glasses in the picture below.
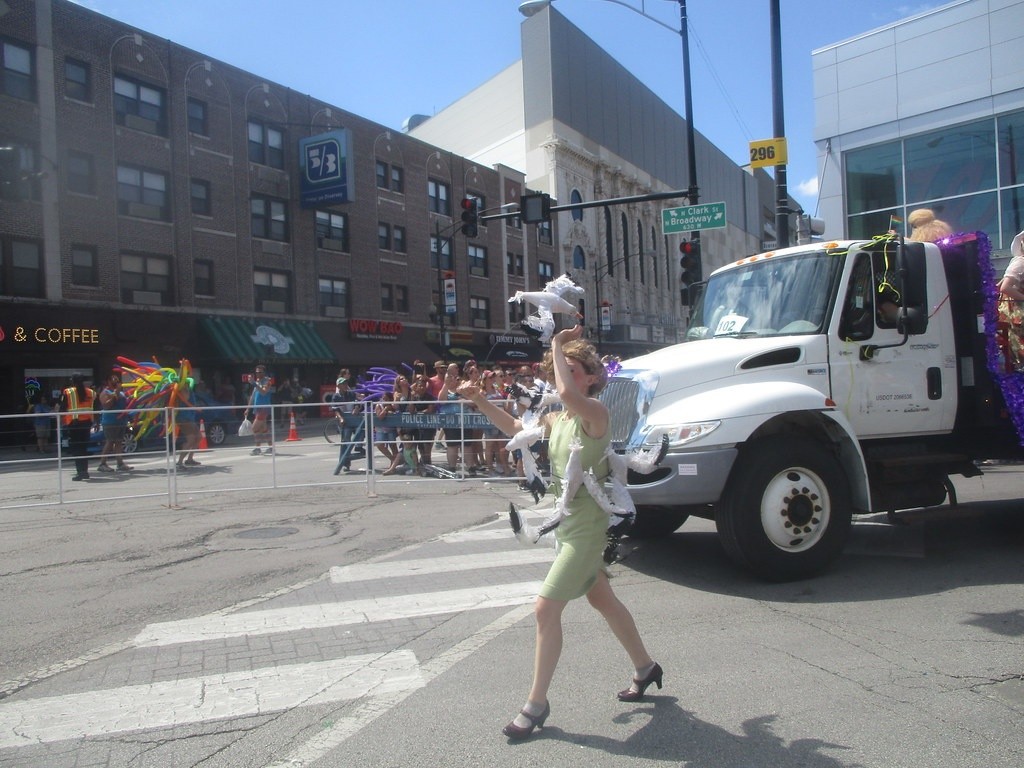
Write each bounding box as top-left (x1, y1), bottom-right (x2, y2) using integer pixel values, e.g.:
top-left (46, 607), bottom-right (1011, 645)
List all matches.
top-left (255, 371), bottom-right (263, 374)
top-left (500, 373), bottom-right (515, 377)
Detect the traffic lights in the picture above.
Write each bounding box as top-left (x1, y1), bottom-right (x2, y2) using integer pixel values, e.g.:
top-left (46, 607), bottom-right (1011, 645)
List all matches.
top-left (681, 288), bottom-right (696, 305)
top-left (460, 197), bottom-right (478, 240)
top-left (679, 240), bottom-right (701, 286)
top-left (798, 215), bottom-right (826, 245)
top-left (520, 194), bottom-right (550, 223)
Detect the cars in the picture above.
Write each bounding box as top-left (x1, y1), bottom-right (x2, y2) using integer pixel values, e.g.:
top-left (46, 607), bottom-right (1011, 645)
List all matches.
top-left (60, 391), bottom-right (239, 457)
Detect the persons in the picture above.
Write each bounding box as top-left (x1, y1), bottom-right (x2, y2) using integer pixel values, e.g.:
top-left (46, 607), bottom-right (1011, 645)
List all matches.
top-left (97, 374), bottom-right (133, 471)
top-left (27, 394), bottom-right (53, 453)
top-left (51, 373), bottom-right (100, 481)
top-left (456, 324), bottom-right (663, 739)
top-left (376, 359), bottom-right (550, 478)
top-left (176, 374), bottom-right (201, 472)
top-left (331, 377), bottom-right (366, 473)
top-left (336, 369), bottom-right (352, 395)
top-left (244, 364), bottom-right (273, 455)
top-left (1001, 230), bottom-right (1024, 302)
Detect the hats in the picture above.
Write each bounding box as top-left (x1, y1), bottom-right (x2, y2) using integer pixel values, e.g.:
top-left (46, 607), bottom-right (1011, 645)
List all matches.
top-left (1010, 231), bottom-right (1024, 256)
top-left (336, 378), bottom-right (347, 386)
top-left (481, 370), bottom-right (496, 382)
top-left (433, 361), bottom-right (448, 368)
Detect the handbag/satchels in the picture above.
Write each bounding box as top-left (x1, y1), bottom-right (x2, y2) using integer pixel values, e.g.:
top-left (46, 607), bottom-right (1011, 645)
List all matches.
top-left (238, 416), bottom-right (255, 436)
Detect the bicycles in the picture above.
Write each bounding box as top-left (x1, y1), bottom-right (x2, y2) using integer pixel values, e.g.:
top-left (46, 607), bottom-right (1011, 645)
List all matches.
top-left (324, 418), bottom-right (343, 445)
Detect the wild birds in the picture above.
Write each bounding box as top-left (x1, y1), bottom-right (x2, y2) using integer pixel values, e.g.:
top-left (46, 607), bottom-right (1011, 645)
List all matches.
top-left (508, 501), bottom-right (561, 547)
top-left (555, 433), bottom-right (670, 565)
top-left (507, 274), bottom-right (585, 342)
top-left (499, 377), bottom-right (567, 506)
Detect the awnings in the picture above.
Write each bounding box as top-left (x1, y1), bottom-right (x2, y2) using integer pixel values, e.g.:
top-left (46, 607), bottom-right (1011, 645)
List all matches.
top-left (0, 303), bottom-right (543, 367)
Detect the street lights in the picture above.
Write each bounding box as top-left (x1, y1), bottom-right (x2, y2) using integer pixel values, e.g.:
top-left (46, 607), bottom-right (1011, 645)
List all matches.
top-left (519, 0), bottom-right (705, 328)
top-left (436, 202), bottom-right (519, 358)
top-left (595, 250), bottom-right (658, 355)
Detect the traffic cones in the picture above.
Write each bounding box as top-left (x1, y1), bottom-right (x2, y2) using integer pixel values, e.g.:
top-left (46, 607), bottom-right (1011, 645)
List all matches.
top-left (286, 412), bottom-right (302, 441)
top-left (197, 420), bottom-right (213, 453)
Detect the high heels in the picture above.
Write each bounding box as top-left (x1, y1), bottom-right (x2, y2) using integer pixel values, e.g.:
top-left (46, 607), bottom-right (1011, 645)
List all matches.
top-left (617, 661), bottom-right (663, 702)
top-left (502, 699), bottom-right (551, 739)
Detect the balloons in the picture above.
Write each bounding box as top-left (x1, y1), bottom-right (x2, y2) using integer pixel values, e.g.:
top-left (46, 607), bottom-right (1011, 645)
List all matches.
top-left (349, 362), bottom-right (440, 415)
top-left (112, 350), bottom-right (204, 441)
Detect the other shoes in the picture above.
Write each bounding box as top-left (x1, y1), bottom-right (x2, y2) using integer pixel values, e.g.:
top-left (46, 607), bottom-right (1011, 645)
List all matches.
top-left (71, 473), bottom-right (89, 481)
top-left (96, 463), bottom-right (114, 472)
top-left (40, 448), bottom-right (53, 454)
top-left (250, 448), bottom-right (262, 455)
top-left (262, 447), bottom-right (275, 454)
top-left (176, 462), bottom-right (188, 471)
top-left (116, 463), bottom-right (134, 470)
top-left (382, 459), bottom-right (530, 493)
top-left (185, 459), bottom-right (202, 465)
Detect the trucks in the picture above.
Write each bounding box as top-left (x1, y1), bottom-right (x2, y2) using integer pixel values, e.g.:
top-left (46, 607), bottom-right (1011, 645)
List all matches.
top-left (595, 231), bottom-right (1023, 581)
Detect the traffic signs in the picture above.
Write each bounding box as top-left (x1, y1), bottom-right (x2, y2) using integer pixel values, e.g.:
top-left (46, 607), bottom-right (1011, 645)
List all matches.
top-left (662, 201), bottom-right (726, 237)
